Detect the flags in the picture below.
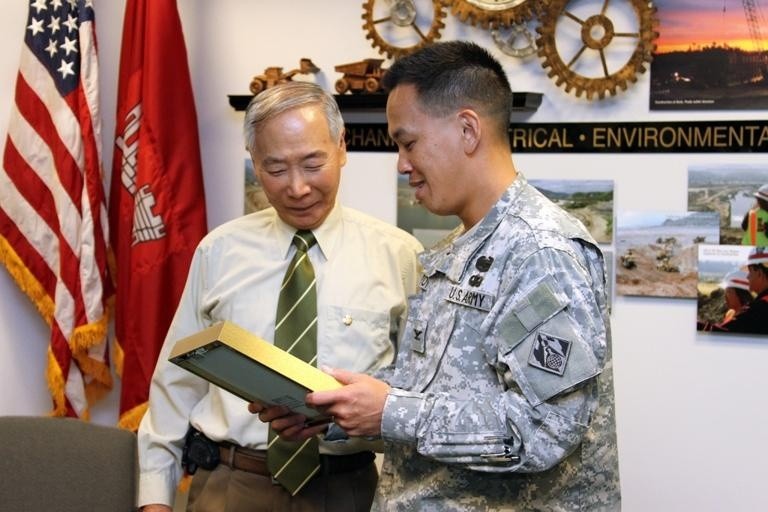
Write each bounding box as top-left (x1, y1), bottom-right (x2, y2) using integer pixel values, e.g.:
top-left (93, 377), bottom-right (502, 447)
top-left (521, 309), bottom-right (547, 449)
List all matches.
top-left (108, 0), bottom-right (208, 435)
top-left (1, 0), bottom-right (117, 417)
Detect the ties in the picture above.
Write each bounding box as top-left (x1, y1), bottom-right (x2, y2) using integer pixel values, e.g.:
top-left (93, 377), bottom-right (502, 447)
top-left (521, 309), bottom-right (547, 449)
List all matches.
top-left (268, 230), bottom-right (323, 497)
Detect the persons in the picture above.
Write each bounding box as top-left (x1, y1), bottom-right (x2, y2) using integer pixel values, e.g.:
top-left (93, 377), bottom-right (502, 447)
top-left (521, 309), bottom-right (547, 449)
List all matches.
top-left (135, 82), bottom-right (427, 512)
top-left (249, 42), bottom-right (622, 512)
top-left (697, 246), bottom-right (767, 335)
top-left (739, 182), bottom-right (768, 245)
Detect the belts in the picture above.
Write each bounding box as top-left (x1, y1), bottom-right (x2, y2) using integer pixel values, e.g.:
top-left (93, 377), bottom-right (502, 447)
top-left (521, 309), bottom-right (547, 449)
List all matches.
top-left (217, 445), bottom-right (375, 479)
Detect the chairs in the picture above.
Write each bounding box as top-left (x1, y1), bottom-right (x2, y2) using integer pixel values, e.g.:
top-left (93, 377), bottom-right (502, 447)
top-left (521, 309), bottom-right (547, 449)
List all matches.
top-left (0, 413), bottom-right (140, 511)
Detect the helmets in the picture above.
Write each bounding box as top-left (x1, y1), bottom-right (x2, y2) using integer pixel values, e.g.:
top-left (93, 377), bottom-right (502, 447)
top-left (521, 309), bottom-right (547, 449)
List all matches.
top-left (720, 267), bottom-right (758, 299)
top-left (740, 246), bottom-right (768, 273)
top-left (753, 184), bottom-right (768, 202)
